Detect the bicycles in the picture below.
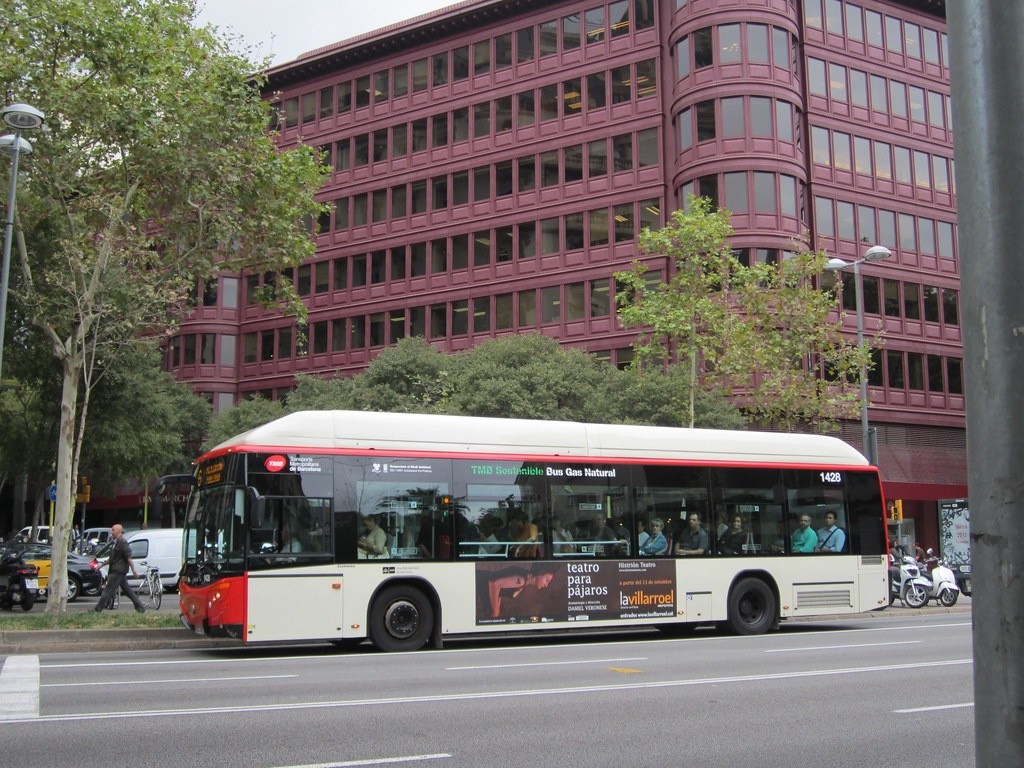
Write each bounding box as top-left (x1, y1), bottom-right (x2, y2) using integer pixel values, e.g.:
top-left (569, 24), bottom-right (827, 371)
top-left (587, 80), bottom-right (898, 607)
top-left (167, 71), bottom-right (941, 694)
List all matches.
top-left (137, 560), bottom-right (163, 610)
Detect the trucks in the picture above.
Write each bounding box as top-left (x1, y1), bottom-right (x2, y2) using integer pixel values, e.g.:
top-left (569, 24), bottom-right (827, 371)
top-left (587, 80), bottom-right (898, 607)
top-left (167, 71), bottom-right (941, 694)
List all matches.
top-left (937, 498), bottom-right (972, 596)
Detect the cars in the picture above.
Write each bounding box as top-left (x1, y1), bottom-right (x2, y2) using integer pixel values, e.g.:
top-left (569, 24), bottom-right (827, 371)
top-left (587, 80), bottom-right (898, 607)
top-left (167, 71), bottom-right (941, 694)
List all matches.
top-left (0, 542), bottom-right (102, 604)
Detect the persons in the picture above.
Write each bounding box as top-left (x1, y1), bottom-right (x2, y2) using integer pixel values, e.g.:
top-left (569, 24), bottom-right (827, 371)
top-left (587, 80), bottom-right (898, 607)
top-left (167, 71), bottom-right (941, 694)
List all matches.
top-left (356, 510), bottom-right (452, 559)
top-left (816, 510), bottom-right (845, 552)
top-left (73, 524), bottom-right (80, 538)
top-left (913, 543), bottom-right (926, 564)
top-left (241, 524), bottom-right (301, 564)
top-left (778, 513), bottom-right (818, 554)
top-left (889, 542), bottom-right (900, 593)
top-left (459, 506), bottom-right (538, 560)
top-left (707, 513), bottom-right (748, 554)
top-left (476, 562), bottom-right (560, 618)
top-left (552, 510), bottom-right (617, 557)
top-left (639, 519), bottom-right (649, 547)
top-left (88, 524), bottom-right (147, 613)
top-left (674, 512), bottom-right (709, 554)
top-left (610, 517), bottom-right (630, 544)
top-left (639, 518), bottom-right (668, 556)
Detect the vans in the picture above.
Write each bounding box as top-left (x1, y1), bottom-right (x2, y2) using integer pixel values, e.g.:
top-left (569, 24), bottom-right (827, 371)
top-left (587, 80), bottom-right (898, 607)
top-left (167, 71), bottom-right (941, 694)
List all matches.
top-left (92, 528), bottom-right (225, 593)
top-left (13, 526), bottom-right (73, 551)
top-left (77, 527), bottom-right (111, 550)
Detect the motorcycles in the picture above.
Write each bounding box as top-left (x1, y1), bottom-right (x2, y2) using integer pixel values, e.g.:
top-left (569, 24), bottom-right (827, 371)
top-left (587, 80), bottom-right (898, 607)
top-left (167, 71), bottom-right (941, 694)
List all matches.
top-left (0, 545), bottom-right (40, 612)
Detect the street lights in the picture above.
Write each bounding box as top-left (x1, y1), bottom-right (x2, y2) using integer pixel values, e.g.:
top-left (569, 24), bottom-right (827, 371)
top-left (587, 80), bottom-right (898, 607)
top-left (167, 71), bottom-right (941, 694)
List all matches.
top-left (823, 246), bottom-right (893, 467)
top-left (1, 101), bottom-right (45, 356)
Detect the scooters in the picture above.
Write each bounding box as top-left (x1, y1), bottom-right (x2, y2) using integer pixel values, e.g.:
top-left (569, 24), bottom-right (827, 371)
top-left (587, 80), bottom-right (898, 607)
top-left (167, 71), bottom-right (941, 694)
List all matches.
top-left (915, 548), bottom-right (960, 607)
top-left (889, 546), bottom-right (934, 609)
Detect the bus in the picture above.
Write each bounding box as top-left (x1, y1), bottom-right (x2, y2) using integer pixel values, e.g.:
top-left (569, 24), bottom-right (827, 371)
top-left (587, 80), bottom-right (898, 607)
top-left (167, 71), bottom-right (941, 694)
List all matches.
top-left (174, 409), bottom-right (893, 653)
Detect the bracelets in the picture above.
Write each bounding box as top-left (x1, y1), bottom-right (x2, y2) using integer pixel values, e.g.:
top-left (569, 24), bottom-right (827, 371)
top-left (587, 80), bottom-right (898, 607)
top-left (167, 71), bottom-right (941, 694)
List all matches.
top-left (102, 562), bottom-right (107, 565)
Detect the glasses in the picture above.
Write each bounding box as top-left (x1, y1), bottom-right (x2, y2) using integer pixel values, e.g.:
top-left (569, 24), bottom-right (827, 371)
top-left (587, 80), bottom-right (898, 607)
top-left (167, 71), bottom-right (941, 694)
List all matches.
top-left (651, 524), bottom-right (661, 528)
